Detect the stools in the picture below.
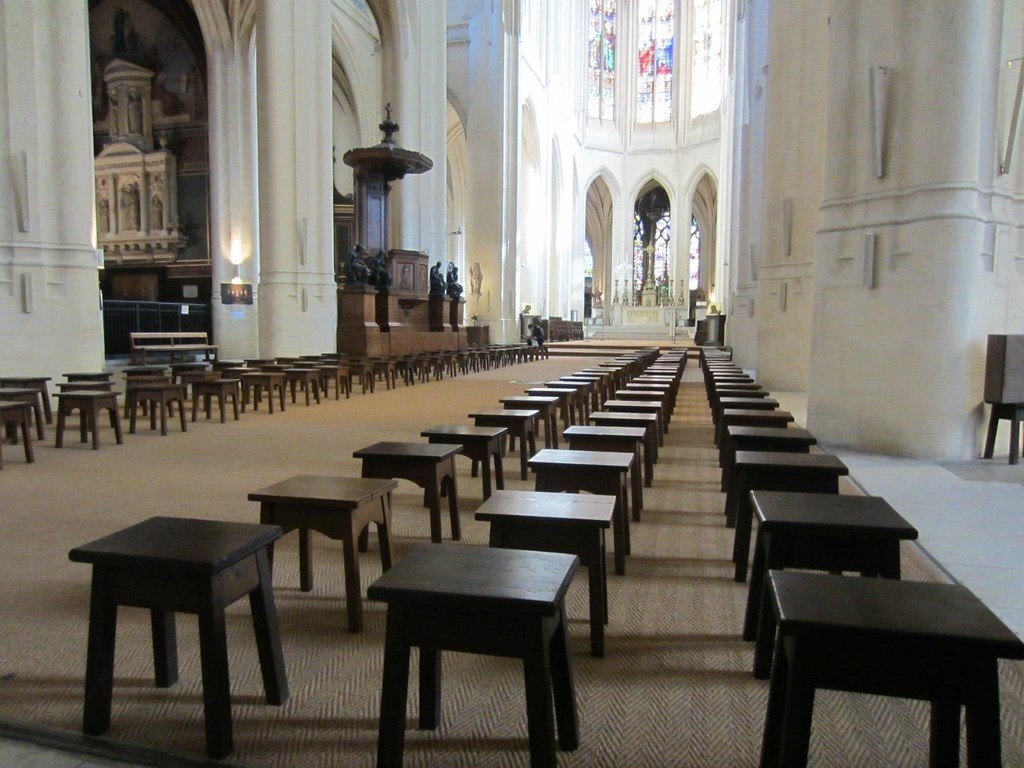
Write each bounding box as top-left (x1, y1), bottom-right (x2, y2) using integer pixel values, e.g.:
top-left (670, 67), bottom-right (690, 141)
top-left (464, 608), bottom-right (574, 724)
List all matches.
top-left (1, 336), bottom-right (1023, 766)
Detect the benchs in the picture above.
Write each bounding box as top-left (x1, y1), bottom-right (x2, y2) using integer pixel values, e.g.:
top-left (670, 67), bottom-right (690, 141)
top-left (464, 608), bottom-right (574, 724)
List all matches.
top-left (130, 332), bottom-right (220, 365)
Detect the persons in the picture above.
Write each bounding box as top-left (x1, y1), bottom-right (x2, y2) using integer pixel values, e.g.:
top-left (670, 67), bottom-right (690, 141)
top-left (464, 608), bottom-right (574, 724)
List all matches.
top-left (124, 184), bottom-right (138, 230)
top-left (523, 305), bottom-right (532, 314)
top-left (431, 262), bottom-right (445, 290)
top-left (100, 201), bottom-right (109, 232)
top-left (527, 324), bottom-right (544, 346)
top-left (348, 245), bottom-right (392, 286)
top-left (152, 197), bottom-right (163, 230)
top-left (710, 305), bottom-right (719, 314)
top-left (447, 267), bottom-right (460, 294)
top-left (109, 98), bottom-right (118, 136)
top-left (128, 93), bottom-right (142, 132)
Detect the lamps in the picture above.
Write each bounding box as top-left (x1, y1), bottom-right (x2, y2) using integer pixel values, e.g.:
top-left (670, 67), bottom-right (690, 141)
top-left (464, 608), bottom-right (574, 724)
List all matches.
top-left (235, 263), bottom-right (241, 277)
top-left (96, 249), bottom-right (105, 269)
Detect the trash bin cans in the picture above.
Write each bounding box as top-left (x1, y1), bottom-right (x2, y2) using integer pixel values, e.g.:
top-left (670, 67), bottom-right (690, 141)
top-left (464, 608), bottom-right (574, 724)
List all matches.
top-left (571, 310), bottom-right (578, 321)
